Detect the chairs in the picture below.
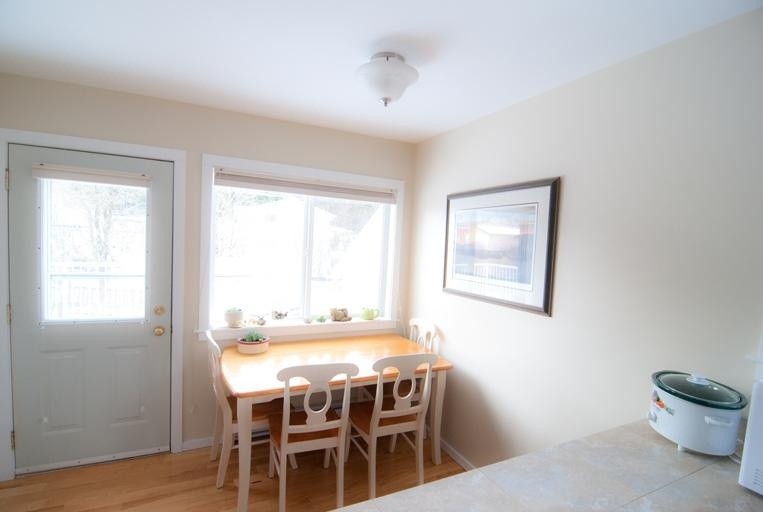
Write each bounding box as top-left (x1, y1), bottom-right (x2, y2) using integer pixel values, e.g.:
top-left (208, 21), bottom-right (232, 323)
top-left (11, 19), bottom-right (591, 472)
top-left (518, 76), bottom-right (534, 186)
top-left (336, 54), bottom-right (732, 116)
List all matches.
top-left (204, 329), bottom-right (300, 490)
top-left (266, 362), bottom-right (361, 510)
top-left (387, 317), bottom-right (438, 455)
top-left (334, 353), bottom-right (438, 499)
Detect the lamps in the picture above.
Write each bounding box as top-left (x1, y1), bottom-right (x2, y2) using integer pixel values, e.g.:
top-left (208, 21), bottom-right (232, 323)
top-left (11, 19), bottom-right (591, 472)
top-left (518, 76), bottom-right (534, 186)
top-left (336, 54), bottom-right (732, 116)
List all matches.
top-left (354, 51), bottom-right (421, 109)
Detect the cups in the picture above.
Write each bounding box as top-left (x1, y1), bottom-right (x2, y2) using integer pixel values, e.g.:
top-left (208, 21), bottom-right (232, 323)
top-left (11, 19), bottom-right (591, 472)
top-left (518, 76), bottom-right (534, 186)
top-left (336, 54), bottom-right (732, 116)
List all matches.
top-left (360, 307), bottom-right (380, 320)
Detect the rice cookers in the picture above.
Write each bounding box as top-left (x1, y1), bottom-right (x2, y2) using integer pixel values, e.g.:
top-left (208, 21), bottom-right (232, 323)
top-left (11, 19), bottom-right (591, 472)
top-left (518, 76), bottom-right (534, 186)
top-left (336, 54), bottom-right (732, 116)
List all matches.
top-left (647, 370), bottom-right (749, 457)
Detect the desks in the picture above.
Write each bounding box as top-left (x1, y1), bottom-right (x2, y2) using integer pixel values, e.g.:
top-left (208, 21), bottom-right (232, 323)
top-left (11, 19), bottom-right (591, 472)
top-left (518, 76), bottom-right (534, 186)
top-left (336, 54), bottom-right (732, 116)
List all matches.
top-left (219, 333), bottom-right (454, 512)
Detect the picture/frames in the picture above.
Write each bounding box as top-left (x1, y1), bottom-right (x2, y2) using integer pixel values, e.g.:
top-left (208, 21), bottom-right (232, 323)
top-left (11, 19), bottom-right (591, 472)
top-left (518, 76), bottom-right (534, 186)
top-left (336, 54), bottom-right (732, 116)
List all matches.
top-left (441, 176), bottom-right (560, 317)
